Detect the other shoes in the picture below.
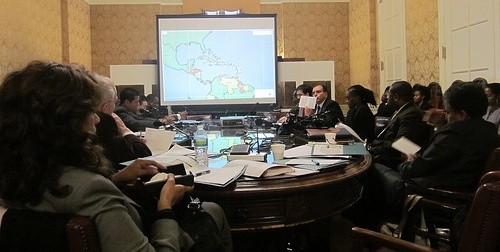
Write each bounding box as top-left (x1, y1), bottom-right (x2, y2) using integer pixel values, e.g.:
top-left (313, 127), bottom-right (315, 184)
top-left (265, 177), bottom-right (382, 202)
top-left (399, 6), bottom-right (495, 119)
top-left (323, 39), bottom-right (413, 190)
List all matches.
top-left (341, 206), bottom-right (360, 223)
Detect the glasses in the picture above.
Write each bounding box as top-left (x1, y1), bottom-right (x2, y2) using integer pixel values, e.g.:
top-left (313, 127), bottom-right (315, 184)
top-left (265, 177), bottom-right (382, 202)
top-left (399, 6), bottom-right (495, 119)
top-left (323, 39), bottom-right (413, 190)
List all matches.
top-left (102, 98), bottom-right (121, 106)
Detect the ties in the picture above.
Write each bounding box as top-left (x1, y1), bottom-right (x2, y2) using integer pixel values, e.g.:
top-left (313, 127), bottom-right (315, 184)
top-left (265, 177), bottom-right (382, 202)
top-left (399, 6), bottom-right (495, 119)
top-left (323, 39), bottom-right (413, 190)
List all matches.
top-left (318, 106), bottom-right (320, 113)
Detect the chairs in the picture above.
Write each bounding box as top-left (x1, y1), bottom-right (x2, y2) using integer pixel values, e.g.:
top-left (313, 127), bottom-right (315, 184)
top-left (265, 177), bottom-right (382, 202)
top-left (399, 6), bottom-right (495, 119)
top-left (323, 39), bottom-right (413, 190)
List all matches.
top-left (0, 210), bottom-right (101, 252)
top-left (403, 147), bottom-right (500, 231)
top-left (351, 171), bottom-right (500, 252)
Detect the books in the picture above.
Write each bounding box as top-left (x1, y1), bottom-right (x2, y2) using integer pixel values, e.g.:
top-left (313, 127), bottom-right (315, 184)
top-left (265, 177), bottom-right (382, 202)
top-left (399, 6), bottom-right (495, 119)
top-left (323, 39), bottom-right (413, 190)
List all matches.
top-left (306, 128), bottom-right (352, 140)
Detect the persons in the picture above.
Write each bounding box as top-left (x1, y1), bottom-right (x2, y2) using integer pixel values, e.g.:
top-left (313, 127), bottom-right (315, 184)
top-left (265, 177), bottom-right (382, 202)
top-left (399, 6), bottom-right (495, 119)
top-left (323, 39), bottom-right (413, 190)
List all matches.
top-left (0, 59), bottom-right (235, 251)
top-left (355, 77), bottom-right (500, 230)
top-left (288, 85), bottom-right (346, 127)
top-left (94, 72), bottom-right (189, 178)
top-left (338, 85), bottom-right (377, 143)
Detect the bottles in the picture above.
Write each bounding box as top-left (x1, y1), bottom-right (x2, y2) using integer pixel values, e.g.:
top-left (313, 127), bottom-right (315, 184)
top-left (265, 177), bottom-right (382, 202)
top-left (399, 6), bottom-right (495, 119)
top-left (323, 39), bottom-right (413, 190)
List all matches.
top-left (193, 125), bottom-right (209, 168)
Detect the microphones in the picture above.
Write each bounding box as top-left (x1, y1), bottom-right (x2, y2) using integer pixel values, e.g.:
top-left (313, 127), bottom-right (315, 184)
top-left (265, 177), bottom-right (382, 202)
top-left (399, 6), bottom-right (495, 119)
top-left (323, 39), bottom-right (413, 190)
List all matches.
top-left (160, 112), bottom-right (193, 149)
top-left (265, 102), bottom-right (275, 113)
top-left (247, 103), bottom-right (260, 127)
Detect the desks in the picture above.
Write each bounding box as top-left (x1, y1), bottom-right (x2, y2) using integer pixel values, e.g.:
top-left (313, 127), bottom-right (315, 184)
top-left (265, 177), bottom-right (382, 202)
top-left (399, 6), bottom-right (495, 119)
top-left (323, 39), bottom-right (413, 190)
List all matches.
top-left (117, 118), bottom-right (373, 252)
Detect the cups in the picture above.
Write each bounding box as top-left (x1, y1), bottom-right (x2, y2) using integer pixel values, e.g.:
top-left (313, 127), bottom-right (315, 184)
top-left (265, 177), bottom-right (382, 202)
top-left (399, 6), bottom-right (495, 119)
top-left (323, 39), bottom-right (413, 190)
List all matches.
top-left (324, 132), bottom-right (337, 148)
top-left (271, 143), bottom-right (285, 162)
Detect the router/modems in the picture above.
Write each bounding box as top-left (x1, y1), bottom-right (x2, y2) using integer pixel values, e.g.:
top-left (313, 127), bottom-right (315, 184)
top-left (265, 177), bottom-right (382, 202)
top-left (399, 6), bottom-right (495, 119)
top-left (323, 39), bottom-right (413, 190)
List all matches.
top-left (230, 143), bottom-right (250, 154)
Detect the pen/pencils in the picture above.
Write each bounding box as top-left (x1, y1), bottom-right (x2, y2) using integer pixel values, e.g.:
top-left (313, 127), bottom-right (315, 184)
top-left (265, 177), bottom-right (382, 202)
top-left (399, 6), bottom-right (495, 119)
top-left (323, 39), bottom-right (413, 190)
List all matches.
top-left (311, 159), bottom-right (320, 166)
top-left (194, 169), bottom-right (213, 177)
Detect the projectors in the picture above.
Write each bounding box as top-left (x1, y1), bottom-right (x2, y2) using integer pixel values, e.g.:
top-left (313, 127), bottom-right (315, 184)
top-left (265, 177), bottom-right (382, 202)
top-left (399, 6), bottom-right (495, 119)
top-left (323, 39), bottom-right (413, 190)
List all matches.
top-left (220, 116), bottom-right (244, 127)
